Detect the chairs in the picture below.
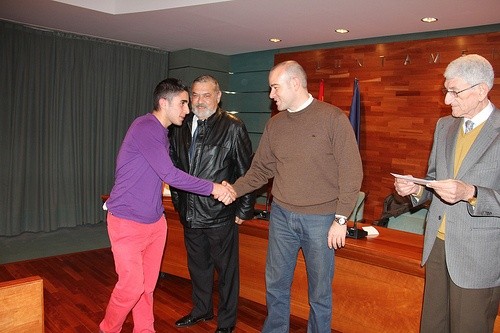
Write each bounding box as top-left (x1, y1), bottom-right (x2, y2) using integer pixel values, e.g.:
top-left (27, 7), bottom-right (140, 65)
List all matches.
top-left (387, 208), bottom-right (428, 235)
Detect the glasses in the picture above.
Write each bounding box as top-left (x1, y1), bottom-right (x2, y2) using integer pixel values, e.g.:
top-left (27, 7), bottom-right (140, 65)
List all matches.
top-left (441, 82), bottom-right (482, 98)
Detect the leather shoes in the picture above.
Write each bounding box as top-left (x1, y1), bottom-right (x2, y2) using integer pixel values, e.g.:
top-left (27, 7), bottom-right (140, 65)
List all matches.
top-left (215, 327), bottom-right (233, 333)
top-left (175, 312), bottom-right (213, 328)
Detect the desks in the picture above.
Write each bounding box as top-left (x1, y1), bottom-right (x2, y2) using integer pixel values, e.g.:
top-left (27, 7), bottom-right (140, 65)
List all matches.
top-left (101, 195), bottom-right (500, 333)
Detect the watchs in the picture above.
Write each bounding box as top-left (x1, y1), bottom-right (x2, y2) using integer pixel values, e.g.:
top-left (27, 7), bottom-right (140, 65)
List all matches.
top-left (334, 216), bottom-right (349, 226)
top-left (467, 185), bottom-right (478, 207)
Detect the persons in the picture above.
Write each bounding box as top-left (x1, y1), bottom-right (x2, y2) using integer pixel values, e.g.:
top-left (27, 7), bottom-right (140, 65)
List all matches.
top-left (98, 77), bottom-right (236, 333)
top-left (165, 76), bottom-right (256, 333)
top-left (395, 55), bottom-right (500, 333)
top-left (215, 60), bottom-right (363, 333)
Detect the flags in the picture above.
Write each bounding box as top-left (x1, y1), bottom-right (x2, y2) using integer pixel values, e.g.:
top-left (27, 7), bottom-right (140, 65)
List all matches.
top-left (319, 82), bottom-right (324, 100)
top-left (348, 78), bottom-right (361, 148)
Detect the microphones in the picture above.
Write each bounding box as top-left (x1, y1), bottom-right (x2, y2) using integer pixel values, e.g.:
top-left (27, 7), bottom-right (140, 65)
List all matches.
top-left (346, 193), bottom-right (369, 239)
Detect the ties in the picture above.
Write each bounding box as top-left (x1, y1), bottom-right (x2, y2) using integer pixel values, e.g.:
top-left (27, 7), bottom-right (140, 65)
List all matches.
top-left (190, 120), bottom-right (204, 165)
top-left (465, 120), bottom-right (475, 134)
